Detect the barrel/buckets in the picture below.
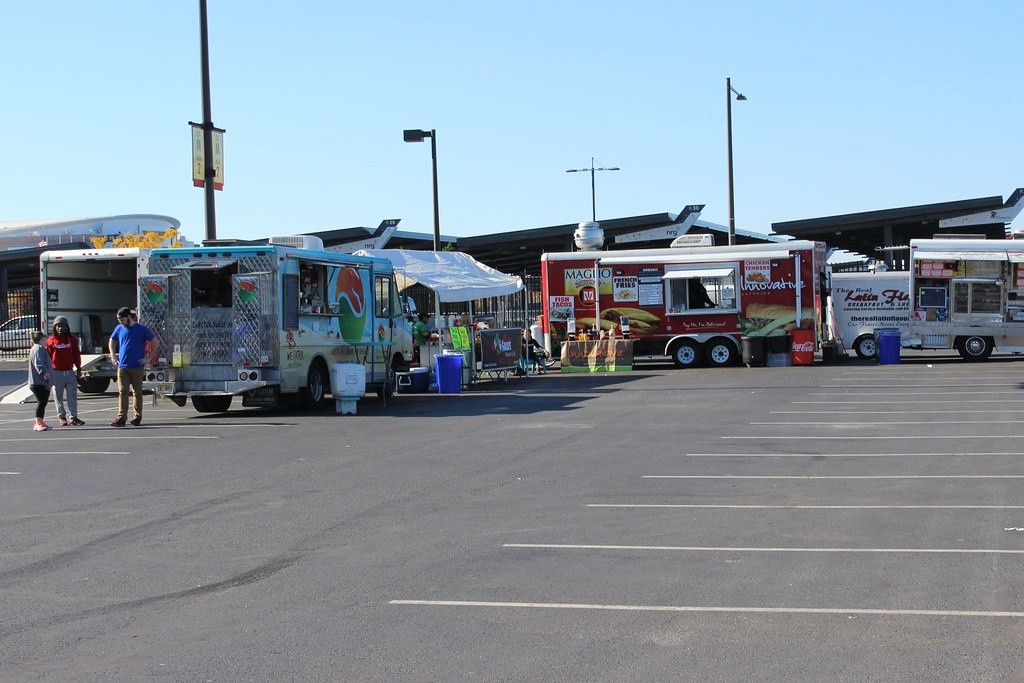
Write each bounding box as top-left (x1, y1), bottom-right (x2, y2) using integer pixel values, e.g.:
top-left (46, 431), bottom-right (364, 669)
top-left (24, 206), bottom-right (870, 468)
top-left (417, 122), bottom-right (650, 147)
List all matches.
top-left (443, 349), bottom-right (472, 390)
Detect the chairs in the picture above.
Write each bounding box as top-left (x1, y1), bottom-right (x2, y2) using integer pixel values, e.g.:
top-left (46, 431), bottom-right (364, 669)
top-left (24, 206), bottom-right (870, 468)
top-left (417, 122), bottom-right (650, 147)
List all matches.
top-left (520, 355), bottom-right (546, 375)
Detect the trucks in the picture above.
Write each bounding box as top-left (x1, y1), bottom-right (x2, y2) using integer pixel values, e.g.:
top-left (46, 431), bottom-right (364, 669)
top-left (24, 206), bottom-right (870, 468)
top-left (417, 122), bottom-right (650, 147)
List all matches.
top-left (830, 272), bottom-right (958, 359)
top-left (541, 233), bottom-right (829, 367)
top-left (39, 247), bottom-right (150, 394)
top-left (907, 239), bottom-right (1024, 363)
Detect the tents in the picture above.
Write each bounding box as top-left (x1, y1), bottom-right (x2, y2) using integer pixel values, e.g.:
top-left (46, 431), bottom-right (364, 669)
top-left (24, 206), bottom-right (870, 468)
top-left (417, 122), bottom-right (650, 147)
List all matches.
top-left (353, 249), bottom-right (529, 383)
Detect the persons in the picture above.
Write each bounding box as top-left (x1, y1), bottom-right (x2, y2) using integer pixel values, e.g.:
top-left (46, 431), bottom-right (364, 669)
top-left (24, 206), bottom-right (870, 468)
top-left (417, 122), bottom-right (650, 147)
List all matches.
top-left (28, 331), bottom-right (53, 430)
top-left (108, 308), bottom-right (158, 427)
top-left (414, 313), bottom-right (431, 363)
top-left (521, 329), bottom-right (549, 374)
top-left (689, 278), bottom-right (716, 309)
top-left (46, 316), bottom-right (85, 425)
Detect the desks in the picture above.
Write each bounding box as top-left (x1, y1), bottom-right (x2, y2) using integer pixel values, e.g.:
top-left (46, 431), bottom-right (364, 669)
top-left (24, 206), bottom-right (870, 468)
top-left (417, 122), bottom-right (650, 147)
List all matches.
top-left (560, 338), bottom-right (641, 373)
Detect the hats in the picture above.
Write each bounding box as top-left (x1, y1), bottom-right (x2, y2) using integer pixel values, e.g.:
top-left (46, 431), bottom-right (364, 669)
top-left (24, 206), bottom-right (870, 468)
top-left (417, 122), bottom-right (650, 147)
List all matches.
top-left (418, 312), bottom-right (430, 320)
top-left (116, 307), bottom-right (136, 319)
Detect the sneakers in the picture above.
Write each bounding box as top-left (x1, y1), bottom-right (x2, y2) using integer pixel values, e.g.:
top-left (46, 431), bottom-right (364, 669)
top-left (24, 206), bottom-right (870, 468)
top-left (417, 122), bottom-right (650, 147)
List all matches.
top-left (34, 420), bottom-right (52, 431)
top-left (130, 416), bottom-right (141, 426)
top-left (110, 418), bottom-right (125, 427)
top-left (69, 418), bottom-right (85, 426)
top-left (60, 417), bottom-right (68, 426)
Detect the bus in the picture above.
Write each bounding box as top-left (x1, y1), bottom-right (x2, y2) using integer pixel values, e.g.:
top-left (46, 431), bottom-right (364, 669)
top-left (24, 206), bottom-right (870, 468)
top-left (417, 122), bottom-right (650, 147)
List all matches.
top-left (141, 235), bottom-right (416, 414)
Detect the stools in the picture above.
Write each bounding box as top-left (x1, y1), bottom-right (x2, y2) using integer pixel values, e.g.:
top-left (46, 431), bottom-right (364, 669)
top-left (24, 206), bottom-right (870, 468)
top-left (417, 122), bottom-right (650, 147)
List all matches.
top-left (334, 398), bottom-right (360, 414)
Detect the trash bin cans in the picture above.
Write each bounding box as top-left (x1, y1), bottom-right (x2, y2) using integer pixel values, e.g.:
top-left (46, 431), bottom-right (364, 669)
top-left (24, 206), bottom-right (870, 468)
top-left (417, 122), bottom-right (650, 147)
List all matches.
top-left (874, 328), bottom-right (899, 364)
top-left (877, 331), bottom-right (902, 364)
top-left (792, 329), bottom-right (814, 366)
top-left (434, 354), bottom-right (464, 394)
top-left (742, 337), bottom-right (769, 369)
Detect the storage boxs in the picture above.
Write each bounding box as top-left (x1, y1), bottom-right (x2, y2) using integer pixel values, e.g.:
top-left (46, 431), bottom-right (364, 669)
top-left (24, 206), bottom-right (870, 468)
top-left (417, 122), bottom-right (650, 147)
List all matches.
top-left (395, 371), bottom-right (429, 394)
top-left (331, 363), bottom-right (366, 397)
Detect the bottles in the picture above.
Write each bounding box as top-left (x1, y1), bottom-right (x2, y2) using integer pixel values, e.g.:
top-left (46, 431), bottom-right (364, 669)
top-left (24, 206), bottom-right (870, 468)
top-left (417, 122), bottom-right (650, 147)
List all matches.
top-left (455, 319), bottom-right (459, 327)
top-left (261, 351), bottom-right (268, 367)
top-left (721, 298), bottom-right (728, 309)
top-left (314, 297), bottom-right (321, 313)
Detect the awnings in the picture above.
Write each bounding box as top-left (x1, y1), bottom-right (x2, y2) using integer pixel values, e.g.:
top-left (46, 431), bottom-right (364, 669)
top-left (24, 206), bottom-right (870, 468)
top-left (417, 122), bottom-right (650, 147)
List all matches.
top-left (912, 251), bottom-right (1008, 261)
top-left (1007, 253), bottom-right (1024, 262)
top-left (657, 269), bottom-right (734, 279)
top-left (172, 260), bottom-right (238, 270)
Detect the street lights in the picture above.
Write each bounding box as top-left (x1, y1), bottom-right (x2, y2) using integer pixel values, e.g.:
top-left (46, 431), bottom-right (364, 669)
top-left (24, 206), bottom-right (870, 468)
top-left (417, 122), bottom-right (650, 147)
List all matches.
top-left (403, 128), bottom-right (442, 250)
top-left (566, 157), bottom-right (620, 222)
top-left (726, 77), bottom-right (747, 245)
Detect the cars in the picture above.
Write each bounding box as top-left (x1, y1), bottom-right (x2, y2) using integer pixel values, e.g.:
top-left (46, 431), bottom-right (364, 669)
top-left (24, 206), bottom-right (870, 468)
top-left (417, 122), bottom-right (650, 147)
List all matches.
top-left (0, 314), bottom-right (35, 351)
